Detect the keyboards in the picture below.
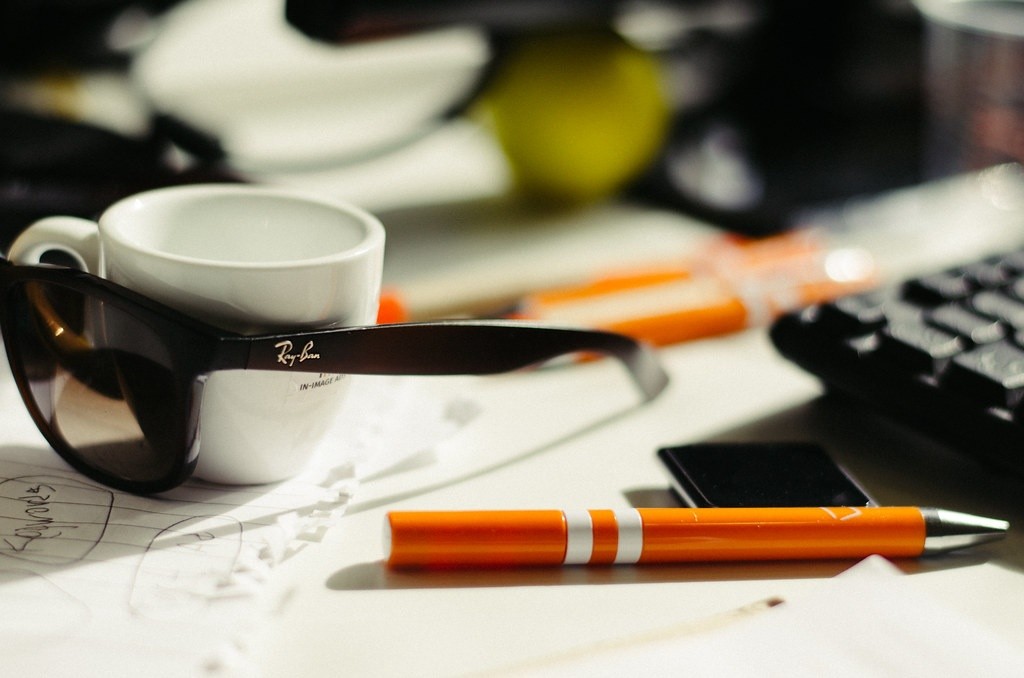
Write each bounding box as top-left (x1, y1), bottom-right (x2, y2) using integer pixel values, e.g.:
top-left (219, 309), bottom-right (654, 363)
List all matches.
top-left (771, 244), bottom-right (1024, 504)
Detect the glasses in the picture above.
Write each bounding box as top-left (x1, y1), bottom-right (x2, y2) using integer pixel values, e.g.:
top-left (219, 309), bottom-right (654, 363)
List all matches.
top-left (0, 256), bottom-right (670, 492)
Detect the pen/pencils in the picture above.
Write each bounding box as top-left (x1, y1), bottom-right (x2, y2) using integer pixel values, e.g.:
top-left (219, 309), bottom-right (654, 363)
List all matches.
top-left (383, 505), bottom-right (1004, 575)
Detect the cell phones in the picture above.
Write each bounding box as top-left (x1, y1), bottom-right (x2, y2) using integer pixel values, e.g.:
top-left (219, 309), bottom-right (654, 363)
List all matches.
top-left (662, 441), bottom-right (877, 510)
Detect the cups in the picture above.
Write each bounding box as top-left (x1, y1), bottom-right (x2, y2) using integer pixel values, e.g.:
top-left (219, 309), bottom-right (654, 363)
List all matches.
top-left (5, 181), bottom-right (386, 486)
